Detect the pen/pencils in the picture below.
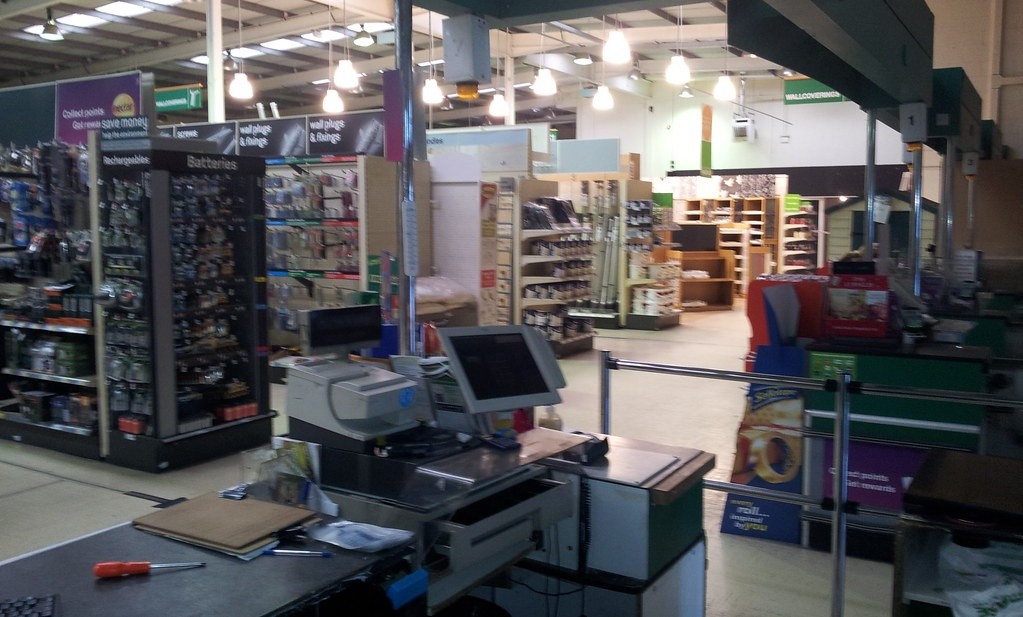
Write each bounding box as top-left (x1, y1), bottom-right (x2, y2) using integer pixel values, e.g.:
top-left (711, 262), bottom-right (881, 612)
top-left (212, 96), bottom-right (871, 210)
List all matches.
top-left (263, 550), bottom-right (333, 558)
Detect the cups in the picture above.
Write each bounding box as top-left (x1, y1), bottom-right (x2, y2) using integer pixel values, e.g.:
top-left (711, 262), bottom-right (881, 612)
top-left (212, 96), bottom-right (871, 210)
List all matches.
top-left (513, 406), bottom-right (535, 433)
top-left (488, 410), bottom-right (514, 436)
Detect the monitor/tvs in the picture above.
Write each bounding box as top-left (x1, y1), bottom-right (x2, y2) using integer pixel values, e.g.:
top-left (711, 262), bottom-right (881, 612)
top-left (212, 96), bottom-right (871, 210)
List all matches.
top-left (890, 280), bottom-right (930, 314)
top-left (437, 325), bottom-right (562, 413)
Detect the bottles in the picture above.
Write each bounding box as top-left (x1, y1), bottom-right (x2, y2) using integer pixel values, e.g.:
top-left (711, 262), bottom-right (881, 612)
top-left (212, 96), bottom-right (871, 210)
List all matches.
top-left (538, 404), bottom-right (563, 431)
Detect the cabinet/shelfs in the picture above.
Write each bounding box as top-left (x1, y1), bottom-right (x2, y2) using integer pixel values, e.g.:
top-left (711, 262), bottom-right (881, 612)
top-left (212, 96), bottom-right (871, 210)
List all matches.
top-left (98, 151), bottom-right (278, 475)
top-left (778, 194), bottom-right (825, 275)
top-left (682, 197), bottom-right (777, 247)
top-left (620, 201), bottom-right (680, 331)
top-left (665, 251), bottom-right (735, 312)
top-left (514, 177), bottom-right (595, 360)
top-left (721, 227), bottom-right (747, 298)
top-left (0, 132), bottom-right (100, 460)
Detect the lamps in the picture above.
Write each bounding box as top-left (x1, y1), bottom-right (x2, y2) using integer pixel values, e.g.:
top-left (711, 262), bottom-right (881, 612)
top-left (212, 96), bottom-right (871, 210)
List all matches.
top-left (679, 4), bottom-right (694, 99)
top-left (530, 24), bottom-right (560, 97)
top-left (666, 0), bottom-right (691, 88)
top-left (715, 45), bottom-right (738, 104)
top-left (335, 0), bottom-right (361, 88)
top-left (421, 9), bottom-right (443, 106)
top-left (39, 5), bottom-right (65, 40)
top-left (628, 65), bottom-right (644, 80)
top-left (228, 0), bottom-right (253, 101)
top-left (601, 7), bottom-right (633, 64)
top-left (591, 66), bottom-right (615, 110)
top-left (574, 51), bottom-right (594, 66)
top-left (491, 29), bottom-right (512, 118)
top-left (323, 2), bottom-right (345, 116)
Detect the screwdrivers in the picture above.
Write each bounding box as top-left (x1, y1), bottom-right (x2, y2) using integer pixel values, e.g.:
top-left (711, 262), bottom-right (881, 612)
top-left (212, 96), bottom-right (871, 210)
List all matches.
top-left (92, 561), bottom-right (208, 577)
